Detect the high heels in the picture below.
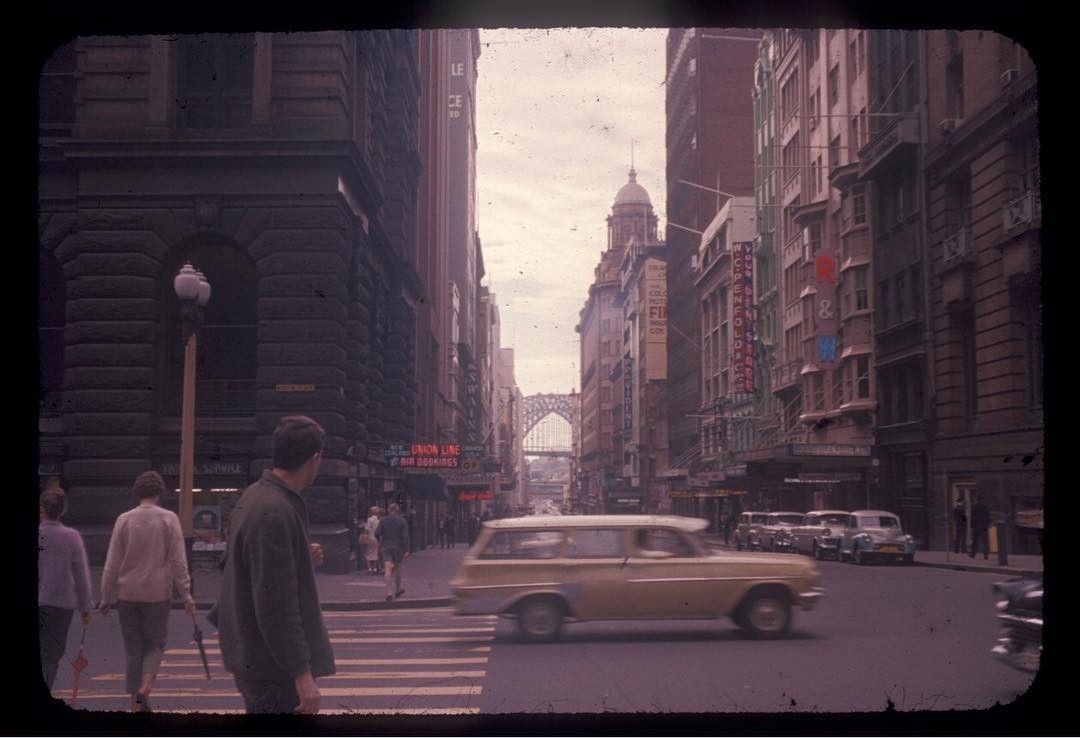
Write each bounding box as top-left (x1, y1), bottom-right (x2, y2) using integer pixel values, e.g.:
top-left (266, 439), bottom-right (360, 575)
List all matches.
top-left (368, 568), bottom-right (372, 573)
top-left (372, 569), bottom-right (377, 573)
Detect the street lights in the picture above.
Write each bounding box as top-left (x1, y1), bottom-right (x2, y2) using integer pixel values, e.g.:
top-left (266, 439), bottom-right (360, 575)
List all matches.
top-left (174, 259), bottom-right (213, 595)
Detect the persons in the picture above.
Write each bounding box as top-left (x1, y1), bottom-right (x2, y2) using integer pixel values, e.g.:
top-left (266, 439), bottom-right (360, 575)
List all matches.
top-left (952, 495), bottom-right (990, 560)
top-left (207, 414), bottom-right (336, 714)
top-left (100, 470), bottom-right (196, 712)
top-left (439, 504), bottom-right (512, 549)
top-left (361, 503), bottom-right (412, 601)
top-left (38, 486), bottom-right (94, 691)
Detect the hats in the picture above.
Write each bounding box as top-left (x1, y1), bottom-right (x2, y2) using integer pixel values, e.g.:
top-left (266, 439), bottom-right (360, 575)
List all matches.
top-left (955, 498), bottom-right (962, 502)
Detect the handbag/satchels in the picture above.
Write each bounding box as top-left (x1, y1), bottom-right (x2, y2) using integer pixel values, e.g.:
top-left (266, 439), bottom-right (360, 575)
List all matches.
top-left (359, 535), bottom-right (370, 544)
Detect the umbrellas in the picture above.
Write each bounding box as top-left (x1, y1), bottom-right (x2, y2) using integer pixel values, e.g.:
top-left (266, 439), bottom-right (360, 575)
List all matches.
top-left (71, 626), bottom-right (88, 707)
top-left (192, 614), bottom-right (211, 680)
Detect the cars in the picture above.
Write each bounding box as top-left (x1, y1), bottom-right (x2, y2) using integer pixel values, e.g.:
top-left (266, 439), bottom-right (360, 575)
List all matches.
top-left (732, 512), bottom-right (768, 552)
top-left (792, 509), bottom-right (852, 561)
top-left (841, 511), bottom-right (917, 566)
top-left (760, 511), bottom-right (804, 553)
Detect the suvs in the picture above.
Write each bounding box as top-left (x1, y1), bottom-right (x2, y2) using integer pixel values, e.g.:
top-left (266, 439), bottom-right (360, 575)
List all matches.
top-left (452, 515), bottom-right (829, 644)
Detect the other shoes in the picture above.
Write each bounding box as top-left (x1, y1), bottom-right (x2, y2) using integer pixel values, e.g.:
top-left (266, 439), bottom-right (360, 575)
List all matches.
top-left (130, 691), bottom-right (151, 713)
top-left (969, 555), bottom-right (975, 559)
top-left (395, 589), bottom-right (405, 597)
top-left (984, 556), bottom-right (988, 560)
top-left (384, 595), bottom-right (391, 601)
